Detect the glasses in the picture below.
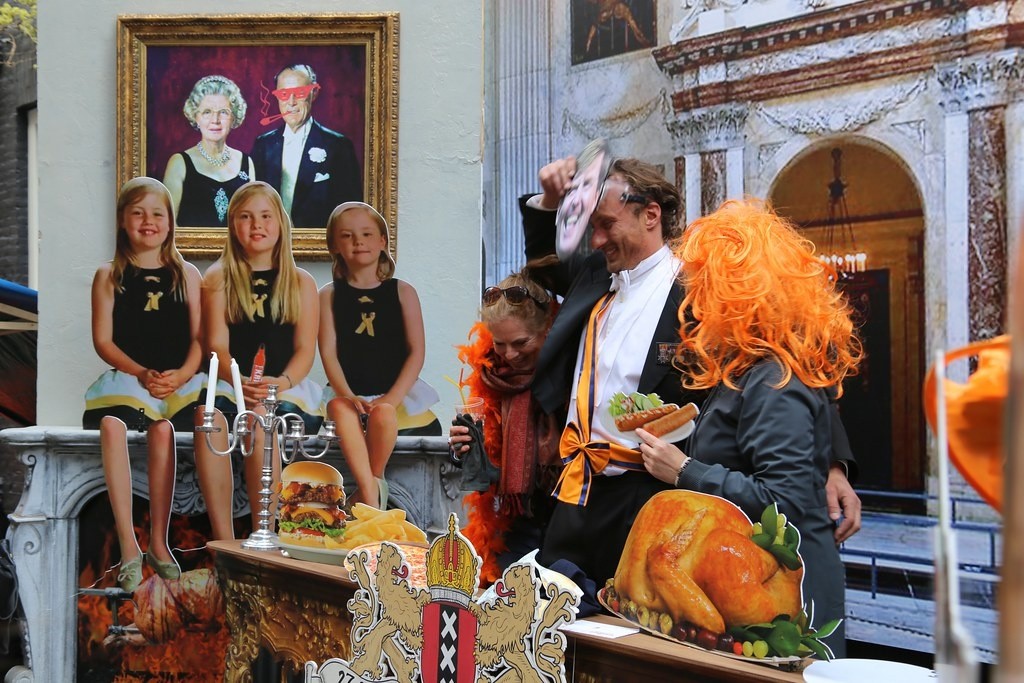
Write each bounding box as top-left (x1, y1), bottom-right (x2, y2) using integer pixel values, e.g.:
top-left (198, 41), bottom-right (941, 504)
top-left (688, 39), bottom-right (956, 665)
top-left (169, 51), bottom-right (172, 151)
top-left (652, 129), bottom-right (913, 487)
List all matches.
top-left (483, 286), bottom-right (547, 311)
top-left (599, 180), bottom-right (648, 215)
top-left (272, 82), bottom-right (319, 100)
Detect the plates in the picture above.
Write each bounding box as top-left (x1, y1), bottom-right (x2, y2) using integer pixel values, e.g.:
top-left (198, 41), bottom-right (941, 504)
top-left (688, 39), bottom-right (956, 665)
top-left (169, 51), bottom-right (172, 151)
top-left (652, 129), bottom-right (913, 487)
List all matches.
top-left (270, 538), bottom-right (348, 566)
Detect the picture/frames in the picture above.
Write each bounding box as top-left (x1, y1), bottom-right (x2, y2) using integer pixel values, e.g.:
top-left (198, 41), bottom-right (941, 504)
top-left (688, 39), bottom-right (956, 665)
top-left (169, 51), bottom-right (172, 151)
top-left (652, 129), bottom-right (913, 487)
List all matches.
top-left (114, 10), bottom-right (401, 259)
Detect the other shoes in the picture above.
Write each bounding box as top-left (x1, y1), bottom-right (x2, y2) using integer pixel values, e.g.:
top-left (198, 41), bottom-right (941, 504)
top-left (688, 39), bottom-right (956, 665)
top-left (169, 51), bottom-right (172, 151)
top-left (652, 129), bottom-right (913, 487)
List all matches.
top-left (375, 476), bottom-right (389, 511)
top-left (118, 551), bottom-right (143, 593)
top-left (146, 546), bottom-right (182, 580)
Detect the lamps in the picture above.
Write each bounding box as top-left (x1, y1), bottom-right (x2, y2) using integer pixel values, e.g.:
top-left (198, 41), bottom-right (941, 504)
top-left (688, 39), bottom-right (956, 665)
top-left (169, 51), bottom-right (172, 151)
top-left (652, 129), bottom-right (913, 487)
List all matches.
top-left (812, 147), bottom-right (869, 275)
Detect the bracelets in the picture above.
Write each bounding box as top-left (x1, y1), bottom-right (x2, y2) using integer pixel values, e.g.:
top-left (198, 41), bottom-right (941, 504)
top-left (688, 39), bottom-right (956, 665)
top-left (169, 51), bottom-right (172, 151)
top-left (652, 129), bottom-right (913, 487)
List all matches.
top-left (281, 374), bottom-right (293, 389)
top-left (676, 457), bottom-right (694, 487)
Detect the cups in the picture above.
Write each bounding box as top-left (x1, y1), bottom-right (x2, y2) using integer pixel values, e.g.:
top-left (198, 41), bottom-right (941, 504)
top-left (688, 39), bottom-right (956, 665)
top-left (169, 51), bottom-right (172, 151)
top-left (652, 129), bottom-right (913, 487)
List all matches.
top-left (453, 396), bottom-right (485, 429)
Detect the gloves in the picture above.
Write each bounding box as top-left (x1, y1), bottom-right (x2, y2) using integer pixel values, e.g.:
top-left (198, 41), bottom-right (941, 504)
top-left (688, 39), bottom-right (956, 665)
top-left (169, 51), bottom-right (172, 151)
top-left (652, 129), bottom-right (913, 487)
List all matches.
top-left (452, 414), bottom-right (501, 495)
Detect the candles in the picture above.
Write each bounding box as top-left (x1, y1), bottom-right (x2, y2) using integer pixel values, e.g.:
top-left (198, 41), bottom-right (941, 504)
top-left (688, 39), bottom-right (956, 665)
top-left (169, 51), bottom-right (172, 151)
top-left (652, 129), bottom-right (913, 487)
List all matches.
top-left (206, 350), bottom-right (219, 412)
top-left (230, 358), bottom-right (246, 417)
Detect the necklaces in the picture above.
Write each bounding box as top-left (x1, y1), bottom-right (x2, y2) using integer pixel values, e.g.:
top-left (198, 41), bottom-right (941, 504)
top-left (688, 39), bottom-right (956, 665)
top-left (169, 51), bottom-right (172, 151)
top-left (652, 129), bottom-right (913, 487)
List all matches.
top-left (196, 141), bottom-right (231, 166)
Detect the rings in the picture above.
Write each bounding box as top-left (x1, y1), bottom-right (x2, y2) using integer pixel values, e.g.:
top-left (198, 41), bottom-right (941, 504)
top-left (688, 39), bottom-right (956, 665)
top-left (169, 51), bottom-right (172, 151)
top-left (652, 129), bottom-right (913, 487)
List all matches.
top-left (447, 437), bottom-right (452, 444)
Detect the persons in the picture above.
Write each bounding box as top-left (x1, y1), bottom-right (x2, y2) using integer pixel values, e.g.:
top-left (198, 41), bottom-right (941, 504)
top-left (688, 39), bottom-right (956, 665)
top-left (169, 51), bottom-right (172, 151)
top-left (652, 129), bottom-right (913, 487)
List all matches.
top-left (518, 156), bottom-right (861, 661)
top-left (317, 202), bottom-right (442, 525)
top-left (555, 137), bottom-right (618, 262)
top-left (447, 273), bottom-right (564, 588)
top-left (161, 74), bottom-right (255, 228)
top-left (250, 65), bottom-right (364, 228)
top-left (634, 205), bottom-right (845, 661)
top-left (83, 176), bottom-right (201, 592)
top-left (196, 181), bottom-right (319, 541)
